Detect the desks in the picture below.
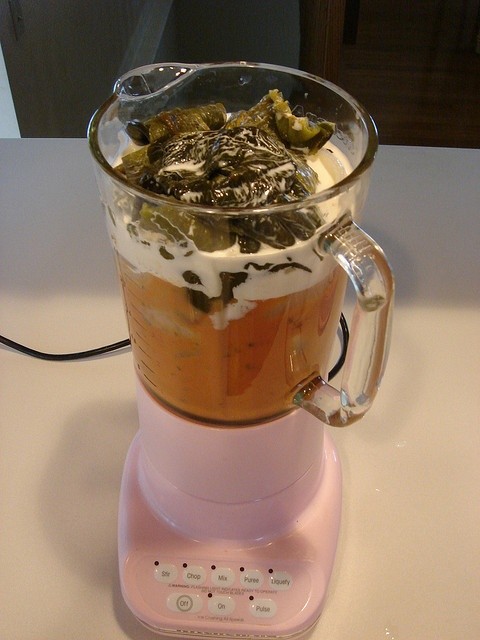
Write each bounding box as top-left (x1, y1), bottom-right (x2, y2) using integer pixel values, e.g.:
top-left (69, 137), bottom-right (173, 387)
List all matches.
top-left (2, 139), bottom-right (479, 637)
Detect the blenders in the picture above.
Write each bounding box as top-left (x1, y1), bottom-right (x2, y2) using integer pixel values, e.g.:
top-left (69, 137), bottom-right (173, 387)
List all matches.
top-left (87, 59), bottom-right (394, 640)
